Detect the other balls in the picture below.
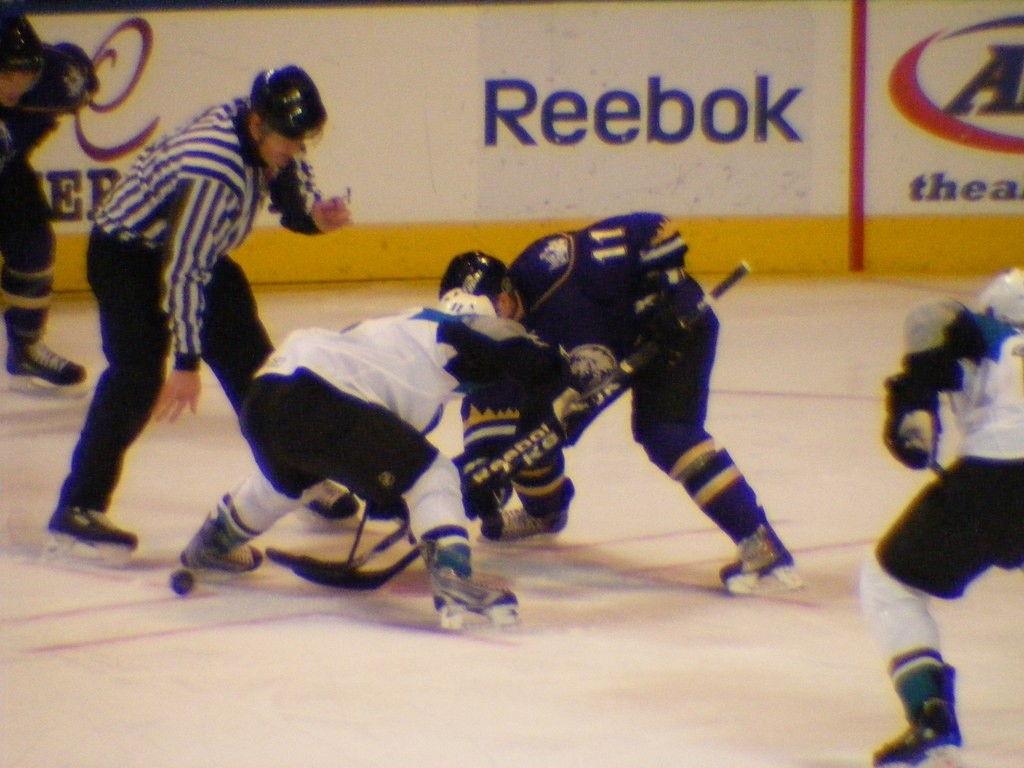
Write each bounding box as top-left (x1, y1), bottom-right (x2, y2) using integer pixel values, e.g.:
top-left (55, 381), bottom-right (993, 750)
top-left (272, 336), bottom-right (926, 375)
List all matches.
top-left (171, 570), bottom-right (195, 596)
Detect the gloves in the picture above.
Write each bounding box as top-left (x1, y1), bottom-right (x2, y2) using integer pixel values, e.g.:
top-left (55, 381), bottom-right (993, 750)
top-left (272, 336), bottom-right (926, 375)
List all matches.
top-left (883, 372), bottom-right (941, 468)
top-left (39, 55), bottom-right (85, 103)
top-left (630, 294), bottom-right (688, 346)
top-left (452, 448), bottom-right (514, 522)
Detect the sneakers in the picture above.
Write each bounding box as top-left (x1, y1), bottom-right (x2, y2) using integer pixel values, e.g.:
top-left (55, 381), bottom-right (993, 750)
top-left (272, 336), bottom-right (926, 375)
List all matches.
top-left (871, 663), bottom-right (962, 768)
top-left (41, 504), bottom-right (137, 564)
top-left (298, 482), bottom-right (362, 532)
top-left (417, 537), bottom-right (519, 628)
top-left (718, 506), bottom-right (803, 596)
top-left (3, 318), bottom-right (87, 400)
top-left (173, 489), bottom-right (264, 583)
top-left (484, 509), bottom-right (568, 542)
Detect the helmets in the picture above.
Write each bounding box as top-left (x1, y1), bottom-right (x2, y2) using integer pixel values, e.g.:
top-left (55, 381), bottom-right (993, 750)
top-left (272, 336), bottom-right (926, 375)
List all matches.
top-left (250, 64), bottom-right (326, 137)
top-left (436, 288), bottom-right (497, 318)
top-left (0, 9), bottom-right (46, 75)
top-left (438, 252), bottom-right (512, 304)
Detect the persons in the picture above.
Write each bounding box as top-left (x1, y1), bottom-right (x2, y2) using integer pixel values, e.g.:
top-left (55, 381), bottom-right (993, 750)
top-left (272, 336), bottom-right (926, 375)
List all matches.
top-left (178, 287), bottom-right (616, 611)
top-left (439, 212), bottom-right (795, 585)
top-left (0, 0), bottom-right (101, 386)
top-left (860, 267), bottom-right (1024, 768)
top-left (47, 64), bottom-right (361, 549)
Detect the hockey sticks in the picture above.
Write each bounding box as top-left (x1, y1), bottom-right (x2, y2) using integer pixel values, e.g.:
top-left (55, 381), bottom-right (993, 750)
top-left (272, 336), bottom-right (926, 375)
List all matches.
top-left (290, 256), bottom-right (756, 593)
top-left (906, 428), bottom-right (947, 478)
top-left (264, 407), bottom-right (572, 571)
